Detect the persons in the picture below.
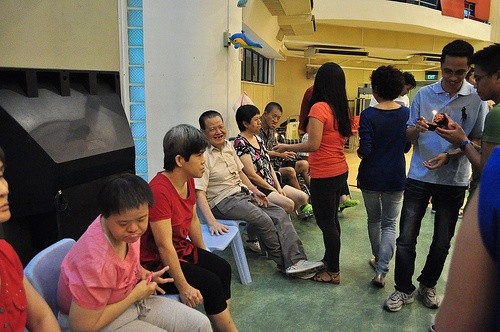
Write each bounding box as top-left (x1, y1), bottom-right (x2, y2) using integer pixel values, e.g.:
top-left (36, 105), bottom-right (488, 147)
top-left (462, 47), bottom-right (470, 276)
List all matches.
top-left (369, 72), bottom-right (417, 107)
top-left (354, 66), bottom-right (412, 287)
top-left (0, 61), bottom-right (358, 332)
top-left (386, 40), bottom-right (500, 332)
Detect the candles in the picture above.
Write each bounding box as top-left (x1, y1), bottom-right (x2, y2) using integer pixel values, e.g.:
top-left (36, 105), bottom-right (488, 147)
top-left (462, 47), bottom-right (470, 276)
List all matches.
top-left (432, 110), bottom-right (436, 122)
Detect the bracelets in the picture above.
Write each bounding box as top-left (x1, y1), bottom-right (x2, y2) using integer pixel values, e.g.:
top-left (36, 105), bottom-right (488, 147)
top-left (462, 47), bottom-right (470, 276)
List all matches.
top-left (445, 152), bottom-right (450, 165)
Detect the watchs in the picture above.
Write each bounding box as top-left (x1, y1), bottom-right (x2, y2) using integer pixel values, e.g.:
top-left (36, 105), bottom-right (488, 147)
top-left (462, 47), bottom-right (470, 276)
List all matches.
top-left (459, 141), bottom-right (473, 151)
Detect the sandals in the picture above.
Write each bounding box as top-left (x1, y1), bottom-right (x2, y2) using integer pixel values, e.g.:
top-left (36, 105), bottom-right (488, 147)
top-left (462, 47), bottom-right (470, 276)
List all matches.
top-left (312, 270), bottom-right (340, 283)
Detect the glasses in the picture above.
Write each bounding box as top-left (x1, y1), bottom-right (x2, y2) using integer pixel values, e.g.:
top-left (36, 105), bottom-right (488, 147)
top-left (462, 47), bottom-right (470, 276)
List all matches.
top-left (440, 65), bottom-right (470, 77)
top-left (472, 71), bottom-right (496, 83)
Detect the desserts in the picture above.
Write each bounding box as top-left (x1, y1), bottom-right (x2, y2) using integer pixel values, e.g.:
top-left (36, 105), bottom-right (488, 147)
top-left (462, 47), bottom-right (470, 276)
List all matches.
top-left (433, 113), bottom-right (448, 128)
top-left (426, 122), bottom-right (437, 131)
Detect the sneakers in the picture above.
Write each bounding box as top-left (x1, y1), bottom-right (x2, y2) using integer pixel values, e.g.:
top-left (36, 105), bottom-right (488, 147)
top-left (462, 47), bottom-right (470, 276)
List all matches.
top-left (418, 284), bottom-right (440, 309)
top-left (384, 291), bottom-right (414, 312)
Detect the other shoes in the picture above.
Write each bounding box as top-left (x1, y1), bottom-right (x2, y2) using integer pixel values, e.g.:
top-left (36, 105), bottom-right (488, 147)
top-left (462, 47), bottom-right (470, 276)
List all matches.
top-left (339, 199), bottom-right (360, 207)
top-left (369, 258), bottom-right (378, 272)
top-left (300, 205), bottom-right (315, 215)
top-left (371, 274), bottom-right (385, 287)
top-left (280, 260), bottom-right (323, 280)
top-left (240, 233), bottom-right (262, 256)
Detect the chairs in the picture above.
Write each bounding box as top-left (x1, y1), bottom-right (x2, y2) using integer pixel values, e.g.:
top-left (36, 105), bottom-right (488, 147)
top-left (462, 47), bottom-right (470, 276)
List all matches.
top-left (24, 201), bottom-right (251, 332)
